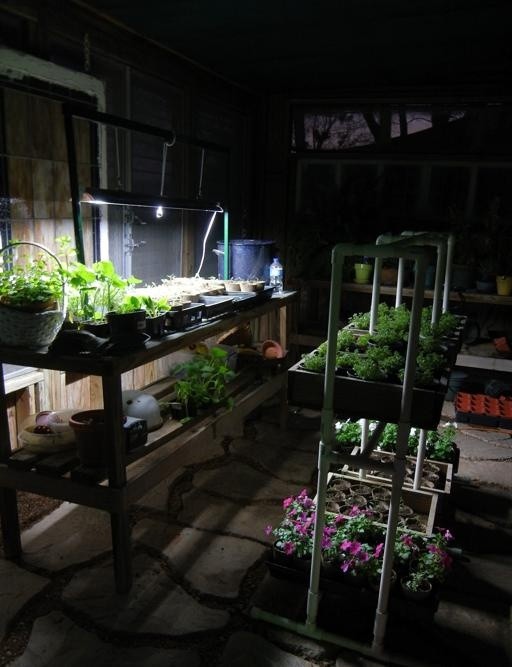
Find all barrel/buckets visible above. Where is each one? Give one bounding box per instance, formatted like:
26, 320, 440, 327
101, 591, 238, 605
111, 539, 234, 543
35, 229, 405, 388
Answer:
212, 238, 273, 285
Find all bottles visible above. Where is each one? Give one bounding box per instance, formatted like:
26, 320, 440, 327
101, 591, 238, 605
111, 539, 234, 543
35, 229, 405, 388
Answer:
269, 258, 283, 294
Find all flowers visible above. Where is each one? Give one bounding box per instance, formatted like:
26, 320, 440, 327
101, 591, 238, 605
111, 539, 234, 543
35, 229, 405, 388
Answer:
265, 490, 453, 586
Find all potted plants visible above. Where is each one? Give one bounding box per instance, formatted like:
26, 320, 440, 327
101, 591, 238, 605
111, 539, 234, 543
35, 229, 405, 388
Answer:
68, 250, 142, 339
142, 295, 170, 338
129, 296, 145, 334
287, 331, 457, 431
107, 303, 148, 350
342, 302, 467, 341
171, 346, 235, 423
335, 419, 458, 474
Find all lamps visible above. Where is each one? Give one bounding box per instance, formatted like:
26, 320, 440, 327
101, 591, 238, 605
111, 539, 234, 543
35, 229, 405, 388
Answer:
156, 137, 174, 220
80, 190, 229, 212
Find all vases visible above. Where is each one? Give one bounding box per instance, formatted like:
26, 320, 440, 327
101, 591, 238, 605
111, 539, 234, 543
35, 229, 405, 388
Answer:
353, 263, 372, 283
382, 264, 398, 286
497, 276, 512, 296
413, 266, 434, 289
140, 281, 267, 310
69, 409, 126, 462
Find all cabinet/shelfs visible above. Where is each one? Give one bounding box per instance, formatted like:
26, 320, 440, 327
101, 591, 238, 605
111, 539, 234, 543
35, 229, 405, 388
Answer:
315, 277, 511, 304
0, 294, 296, 588
456, 353, 509, 372
